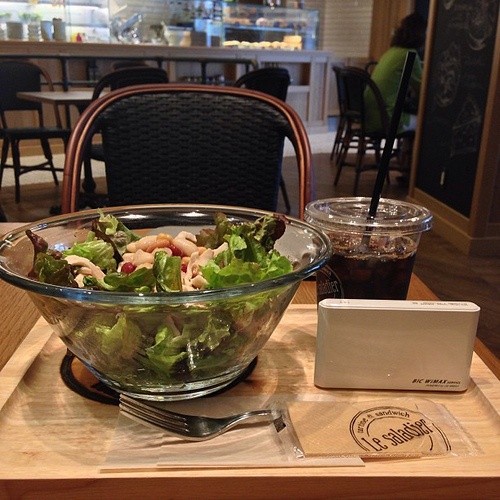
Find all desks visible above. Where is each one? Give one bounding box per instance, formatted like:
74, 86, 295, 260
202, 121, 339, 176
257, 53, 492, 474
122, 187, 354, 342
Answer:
16, 89, 92, 129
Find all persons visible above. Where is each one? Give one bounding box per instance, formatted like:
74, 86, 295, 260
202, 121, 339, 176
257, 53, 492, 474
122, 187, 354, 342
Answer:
365, 13, 426, 192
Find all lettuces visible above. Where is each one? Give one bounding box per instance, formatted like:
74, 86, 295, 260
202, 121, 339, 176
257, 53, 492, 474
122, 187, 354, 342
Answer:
26, 209, 293, 384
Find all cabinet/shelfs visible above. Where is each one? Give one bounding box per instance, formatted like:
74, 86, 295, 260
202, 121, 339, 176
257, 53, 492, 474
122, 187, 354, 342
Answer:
0, 40, 333, 157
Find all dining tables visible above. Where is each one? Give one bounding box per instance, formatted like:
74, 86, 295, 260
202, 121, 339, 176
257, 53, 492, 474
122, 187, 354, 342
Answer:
0, 224, 500, 500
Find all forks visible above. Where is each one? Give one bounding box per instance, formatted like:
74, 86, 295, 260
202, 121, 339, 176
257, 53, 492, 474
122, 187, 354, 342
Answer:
119, 394, 287, 441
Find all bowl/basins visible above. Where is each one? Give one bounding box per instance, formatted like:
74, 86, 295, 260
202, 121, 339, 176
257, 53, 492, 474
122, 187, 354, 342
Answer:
0, 204, 333, 401
164, 27, 184, 47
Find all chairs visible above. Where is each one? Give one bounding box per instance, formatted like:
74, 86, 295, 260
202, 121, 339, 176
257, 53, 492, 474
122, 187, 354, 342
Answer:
0, 58, 417, 221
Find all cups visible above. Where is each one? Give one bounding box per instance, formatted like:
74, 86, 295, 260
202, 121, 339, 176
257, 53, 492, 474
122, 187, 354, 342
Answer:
6, 22, 23, 40
40, 20, 52, 40
305, 197, 436, 314
284, 36, 301, 50
54, 22, 65, 40
195, 19, 222, 48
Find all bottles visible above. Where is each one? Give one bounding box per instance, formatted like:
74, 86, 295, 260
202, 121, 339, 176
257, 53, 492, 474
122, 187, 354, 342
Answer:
71, 33, 86, 42
